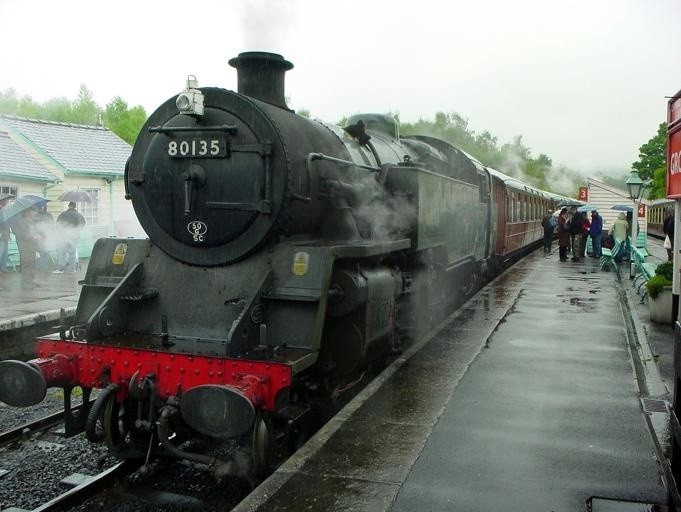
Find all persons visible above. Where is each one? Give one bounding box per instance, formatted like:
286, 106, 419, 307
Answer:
0, 187, 93, 284
541, 198, 635, 262
663, 208, 675, 262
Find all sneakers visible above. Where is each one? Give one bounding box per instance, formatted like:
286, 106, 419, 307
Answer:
587, 252, 601, 258
560, 249, 579, 262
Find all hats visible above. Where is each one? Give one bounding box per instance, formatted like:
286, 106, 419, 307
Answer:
591, 208, 596, 212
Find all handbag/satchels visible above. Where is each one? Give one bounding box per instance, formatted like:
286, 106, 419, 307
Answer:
664, 235, 671, 249
603, 235, 613, 248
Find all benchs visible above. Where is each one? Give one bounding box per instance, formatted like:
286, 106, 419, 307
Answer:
586, 231, 663, 304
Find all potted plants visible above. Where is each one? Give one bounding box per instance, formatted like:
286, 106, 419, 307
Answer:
646, 259, 674, 324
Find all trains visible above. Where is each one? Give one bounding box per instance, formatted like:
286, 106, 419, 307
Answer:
1, 46, 587, 485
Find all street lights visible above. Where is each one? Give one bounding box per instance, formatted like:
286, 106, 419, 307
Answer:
624, 168, 643, 279
